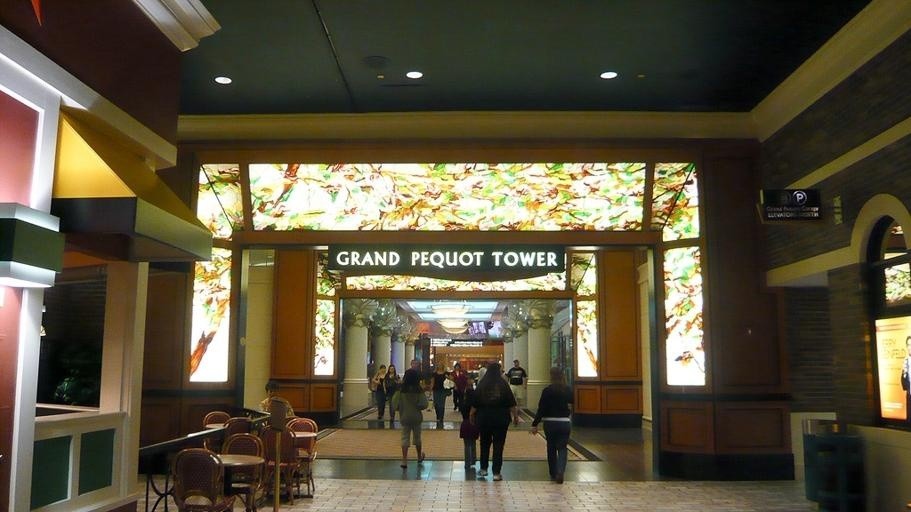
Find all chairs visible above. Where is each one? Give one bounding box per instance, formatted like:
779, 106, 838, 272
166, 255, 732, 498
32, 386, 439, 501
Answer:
166, 410, 319, 512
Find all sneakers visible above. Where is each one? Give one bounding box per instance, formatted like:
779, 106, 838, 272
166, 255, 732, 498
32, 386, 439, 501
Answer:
465, 457, 475, 468
378, 413, 394, 422
454, 403, 458, 410
437, 416, 443, 420
478, 469, 488, 476
493, 474, 502, 480
552, 474, 563, 483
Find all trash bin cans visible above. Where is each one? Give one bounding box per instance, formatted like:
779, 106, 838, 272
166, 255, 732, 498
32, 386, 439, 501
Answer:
801, 419, 866, 512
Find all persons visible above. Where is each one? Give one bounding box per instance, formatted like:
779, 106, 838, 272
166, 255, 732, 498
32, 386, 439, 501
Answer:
410, 359, 528, 432
372, 365, 387, 423
257, 382, 304, 479
392, 369, 428, 468
530, 366, 574, 484
901, 337, 911, 427
459, 391, 479, 468
382, 365, 401, 424
469, 363, 520, 481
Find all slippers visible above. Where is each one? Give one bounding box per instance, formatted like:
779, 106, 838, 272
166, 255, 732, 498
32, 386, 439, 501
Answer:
400, 465, 407, 468
418, 452, 425, 462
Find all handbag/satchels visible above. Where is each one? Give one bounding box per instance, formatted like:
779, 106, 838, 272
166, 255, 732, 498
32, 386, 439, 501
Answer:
443, 379, 455, 389
